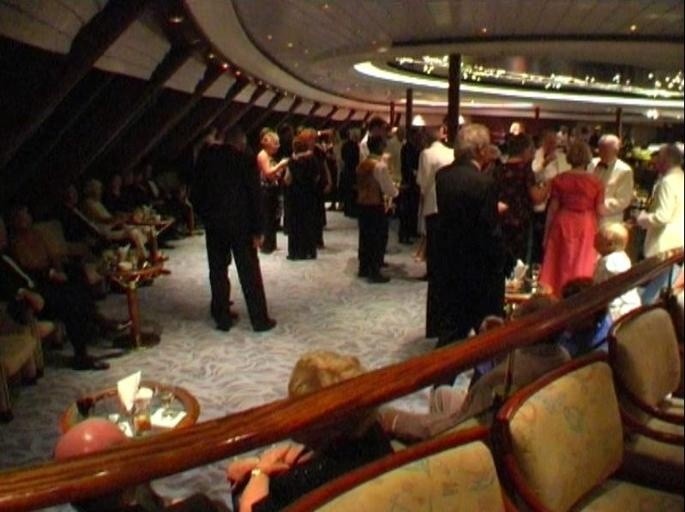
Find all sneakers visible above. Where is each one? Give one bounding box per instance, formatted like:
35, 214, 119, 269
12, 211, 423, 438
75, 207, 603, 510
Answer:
215, 312, 278, 334
356, 261, 391, 285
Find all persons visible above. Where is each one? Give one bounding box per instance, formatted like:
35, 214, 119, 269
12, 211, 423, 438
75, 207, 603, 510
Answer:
55, 293, 572, 511
193, 113, 684, 391
0, 162, 194, 371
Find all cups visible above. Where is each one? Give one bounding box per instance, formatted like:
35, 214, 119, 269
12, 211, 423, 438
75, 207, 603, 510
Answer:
158, 383, 175, 415
131, 399, 154, 438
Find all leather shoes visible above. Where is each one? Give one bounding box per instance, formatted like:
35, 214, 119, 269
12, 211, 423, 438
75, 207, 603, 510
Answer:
96, 317, 133, 338
70, 353, 111, 372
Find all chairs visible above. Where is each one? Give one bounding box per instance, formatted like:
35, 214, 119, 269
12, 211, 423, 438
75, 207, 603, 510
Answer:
37, 219, 67, 263
0, 302, 46, 421
